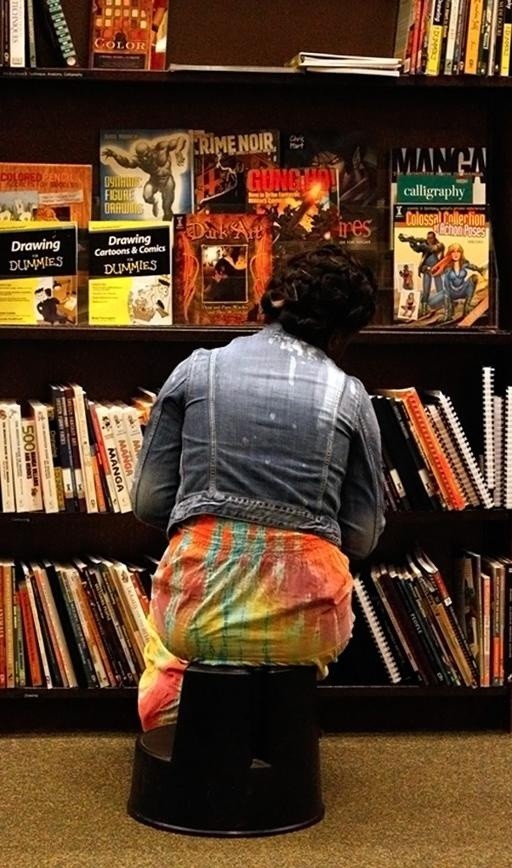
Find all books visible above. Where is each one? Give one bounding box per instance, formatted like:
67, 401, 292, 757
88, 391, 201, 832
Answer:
87, 125, 494, 331
0, 162, 93, 325
354, 551, 512, 691
0, 383, 160, 513
369, 367, 512, 510
1, 0, 169, 71
392, 0, 511, 77
170, 52, 401, 78
0, 554, 159, 688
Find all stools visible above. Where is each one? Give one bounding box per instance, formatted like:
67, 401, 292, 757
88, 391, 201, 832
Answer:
126, 661, 324, 838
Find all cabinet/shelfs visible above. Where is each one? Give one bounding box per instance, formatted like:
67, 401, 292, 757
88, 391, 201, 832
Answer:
1, 67, 512, 736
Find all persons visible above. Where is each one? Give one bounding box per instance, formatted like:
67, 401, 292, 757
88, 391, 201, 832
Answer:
133, 246, 386, 730
